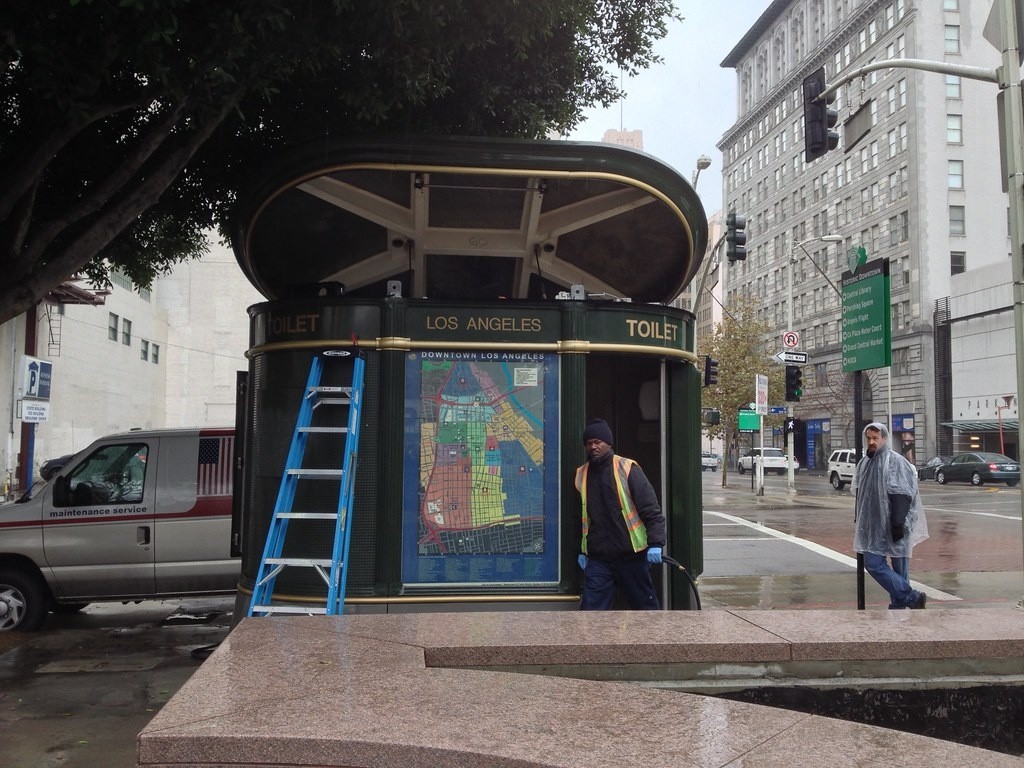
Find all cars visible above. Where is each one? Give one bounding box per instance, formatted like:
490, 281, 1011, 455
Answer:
918, 452, 1021, 487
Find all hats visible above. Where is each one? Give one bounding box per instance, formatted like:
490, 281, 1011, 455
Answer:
581, 416, 614, 449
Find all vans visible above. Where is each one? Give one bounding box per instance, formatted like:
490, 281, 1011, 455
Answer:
0, 427, 243, 631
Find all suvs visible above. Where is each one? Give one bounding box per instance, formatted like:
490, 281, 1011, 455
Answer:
737, 448, 800, 476
826, 448, 866, 490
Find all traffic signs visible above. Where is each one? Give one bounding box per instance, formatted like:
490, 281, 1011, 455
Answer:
770, 351, 808, 366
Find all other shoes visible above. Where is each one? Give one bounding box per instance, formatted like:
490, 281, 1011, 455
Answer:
914, 592, 926, 609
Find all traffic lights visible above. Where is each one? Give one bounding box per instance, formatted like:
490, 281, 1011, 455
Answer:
704, 358, 718, 386
785, 365, 802, 402
803, 66, 839, 163
706, 411, 720, 425
786, 418, 796, 433
726, 207, 747, 267
784, 234, 843, 495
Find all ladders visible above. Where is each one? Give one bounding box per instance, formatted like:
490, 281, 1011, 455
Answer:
246, 349, 367, 615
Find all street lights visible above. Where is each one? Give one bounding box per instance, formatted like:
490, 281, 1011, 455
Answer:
692, 157, 712, 193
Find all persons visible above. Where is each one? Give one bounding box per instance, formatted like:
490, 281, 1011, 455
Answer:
567, 417, 665, 610
856, 423, 929, 610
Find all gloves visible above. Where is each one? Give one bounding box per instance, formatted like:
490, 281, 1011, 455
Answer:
646, 547, 663, 564
578, 552, 588, 571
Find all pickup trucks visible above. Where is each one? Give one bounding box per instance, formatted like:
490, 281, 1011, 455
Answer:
702, 454, 717, 472
